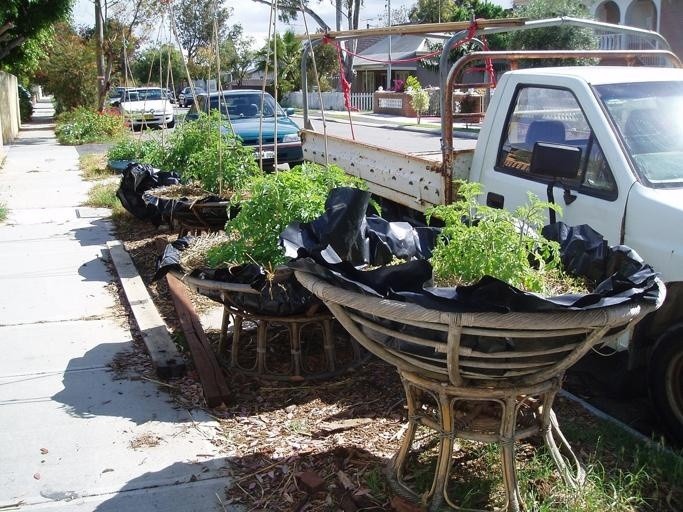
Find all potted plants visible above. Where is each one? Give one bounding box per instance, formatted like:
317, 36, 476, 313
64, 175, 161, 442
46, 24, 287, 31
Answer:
105, 105, 666, 512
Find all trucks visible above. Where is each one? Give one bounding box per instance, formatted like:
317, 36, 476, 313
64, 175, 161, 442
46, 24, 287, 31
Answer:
301, 49, 682, 434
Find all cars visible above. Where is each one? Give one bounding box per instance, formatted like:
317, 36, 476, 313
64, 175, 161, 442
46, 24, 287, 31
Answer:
104, 87, 175, 131
182, 89, 304, 173
178, 87, 206, 107
